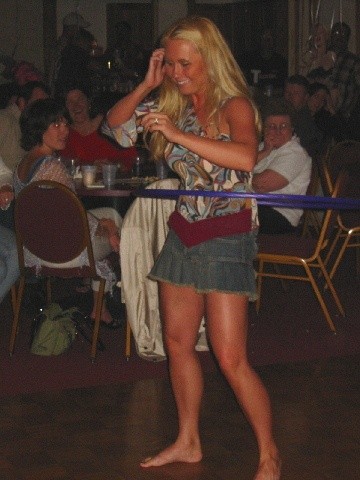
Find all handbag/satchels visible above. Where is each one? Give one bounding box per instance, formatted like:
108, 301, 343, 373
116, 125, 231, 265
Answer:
28, 300, 106, 358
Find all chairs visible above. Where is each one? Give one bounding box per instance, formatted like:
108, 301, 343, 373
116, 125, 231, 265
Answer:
119, 178, 210, 362
7, 180, 133, 362
254, 139, 360, 332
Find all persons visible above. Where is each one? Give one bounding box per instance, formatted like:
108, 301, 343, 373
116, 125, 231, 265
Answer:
12, 99, 124, 330
103, 17, 283, 480
55, 83, 138, 176
0, 159, 26, 304
0, 81, 49, 172
37, 12, 148, 122
251, 110, 313, 236
238, 21, 360, 159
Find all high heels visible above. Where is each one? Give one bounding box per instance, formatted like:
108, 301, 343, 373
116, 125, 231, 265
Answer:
90, 317, 123, 330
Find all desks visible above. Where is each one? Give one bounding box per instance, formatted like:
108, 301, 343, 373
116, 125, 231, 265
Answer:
75, 188, 134, 198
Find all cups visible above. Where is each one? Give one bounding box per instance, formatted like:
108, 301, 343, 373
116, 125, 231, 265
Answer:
53, 151, 64, 163
67, 159, 81, 177
81, 165, 97, 187
101, 164, 118, 186
129, 152, 144, 177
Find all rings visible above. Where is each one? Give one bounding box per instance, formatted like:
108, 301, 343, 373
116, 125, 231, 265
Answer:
154, 117, 158, 124
5, 199, 9, 201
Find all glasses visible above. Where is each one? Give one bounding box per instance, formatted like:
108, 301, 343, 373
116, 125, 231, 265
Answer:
48, 122, 70, 128
332, 30, 350, 37
265, 122, 290, 129
